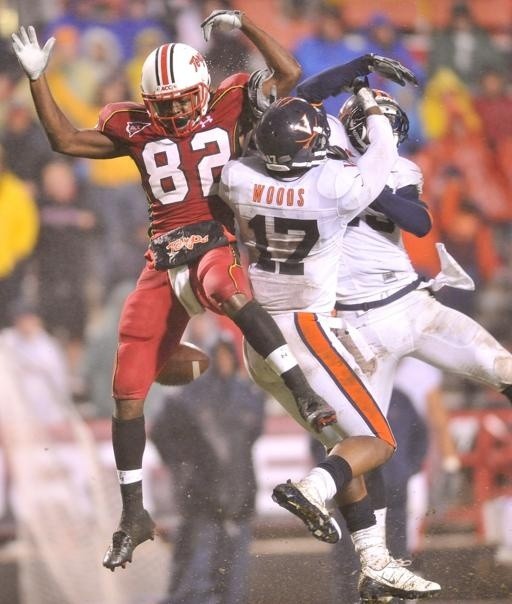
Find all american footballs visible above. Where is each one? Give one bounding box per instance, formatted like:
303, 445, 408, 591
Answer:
155, 341, 211, 385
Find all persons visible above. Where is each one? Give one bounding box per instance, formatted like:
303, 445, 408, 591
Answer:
0, 0, 512, 604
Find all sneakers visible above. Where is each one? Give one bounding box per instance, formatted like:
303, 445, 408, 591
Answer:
359, 556, 441, 604
272, 479, 342, 544
300, 395, 337, 430
103, 510, 156, 571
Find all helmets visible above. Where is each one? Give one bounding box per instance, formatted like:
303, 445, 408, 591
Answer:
338, 89, 409, 154
256, 96, 325, 172
141, 43, 211, 136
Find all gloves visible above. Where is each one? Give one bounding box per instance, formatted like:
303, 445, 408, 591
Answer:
201, 10, 245, 41
371, 53, 418, 86
247, 69, 277, 119
12, 25, 55, 80
